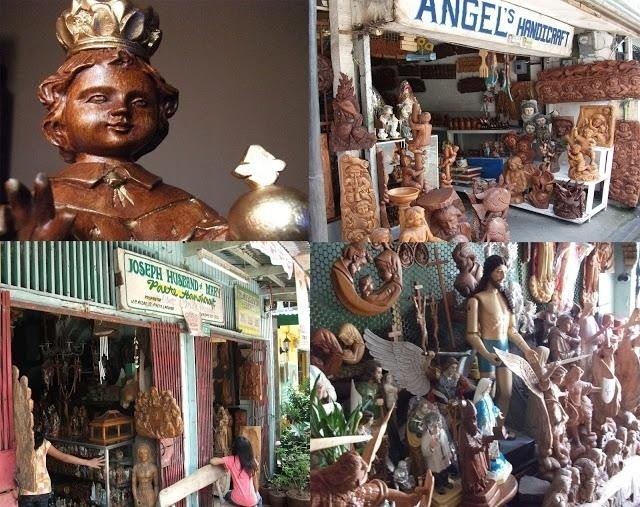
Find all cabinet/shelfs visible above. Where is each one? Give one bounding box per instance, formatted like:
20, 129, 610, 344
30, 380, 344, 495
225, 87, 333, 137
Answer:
43, 433, 133, 507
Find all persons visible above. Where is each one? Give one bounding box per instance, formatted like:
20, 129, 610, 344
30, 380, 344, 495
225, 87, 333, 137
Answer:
0, 0, 228, 238
312, 55, 640, 507
210, 436, 264, 507
20, 428, 108, 505
129, 442, 159, 506
